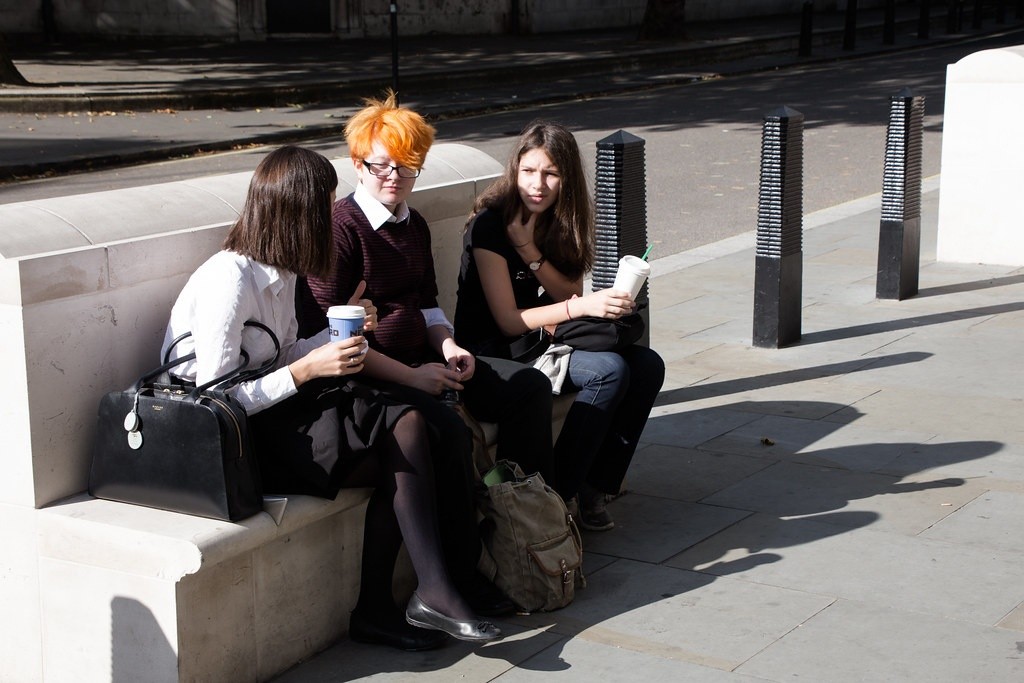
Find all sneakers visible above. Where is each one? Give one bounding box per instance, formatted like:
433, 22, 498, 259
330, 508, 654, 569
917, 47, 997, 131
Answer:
577, 488, 614, 530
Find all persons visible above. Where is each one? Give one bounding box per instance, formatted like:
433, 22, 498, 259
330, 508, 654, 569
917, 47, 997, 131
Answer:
160, 146, 503, 649
453, 124, 668, 530
293, 97, 553, 616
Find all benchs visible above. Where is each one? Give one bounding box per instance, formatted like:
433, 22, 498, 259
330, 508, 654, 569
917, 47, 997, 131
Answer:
1, 142, 632, 683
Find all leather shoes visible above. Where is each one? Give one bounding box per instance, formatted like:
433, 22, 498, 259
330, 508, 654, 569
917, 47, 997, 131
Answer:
405, 594, 503, 641
349, 613, 446, 651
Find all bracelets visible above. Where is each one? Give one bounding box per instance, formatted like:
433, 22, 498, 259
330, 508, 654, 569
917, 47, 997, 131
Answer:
565, 299, 571, 320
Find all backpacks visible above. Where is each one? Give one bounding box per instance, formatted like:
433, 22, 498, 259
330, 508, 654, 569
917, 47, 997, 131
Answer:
478, 460, 586, 612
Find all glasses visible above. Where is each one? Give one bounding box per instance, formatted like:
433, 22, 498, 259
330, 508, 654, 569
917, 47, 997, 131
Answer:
362, 161, 420, 178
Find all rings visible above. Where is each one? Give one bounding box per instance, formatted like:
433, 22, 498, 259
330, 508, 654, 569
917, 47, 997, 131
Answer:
351, 358, 355, 366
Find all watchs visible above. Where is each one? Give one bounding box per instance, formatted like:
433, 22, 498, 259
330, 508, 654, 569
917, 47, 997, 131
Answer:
528, 255, 546, 271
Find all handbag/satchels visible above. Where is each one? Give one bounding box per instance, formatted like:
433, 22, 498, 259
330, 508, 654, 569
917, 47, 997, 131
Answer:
89, 381, 264, 522
552, 314, 645, 352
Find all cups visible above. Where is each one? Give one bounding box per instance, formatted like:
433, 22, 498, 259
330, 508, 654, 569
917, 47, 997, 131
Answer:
614, 255, 652, 308
327, 304, 367, 342
